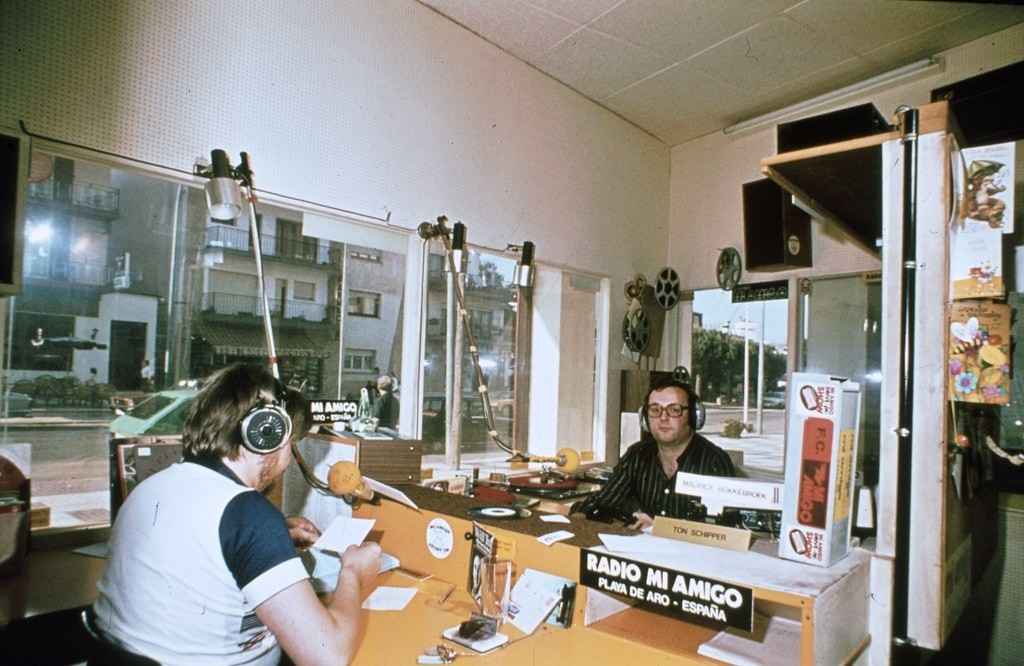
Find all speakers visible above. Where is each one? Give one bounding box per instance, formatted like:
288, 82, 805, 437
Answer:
743, 177, 812, 273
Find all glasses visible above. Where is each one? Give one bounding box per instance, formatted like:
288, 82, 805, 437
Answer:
646, 400, 693, 426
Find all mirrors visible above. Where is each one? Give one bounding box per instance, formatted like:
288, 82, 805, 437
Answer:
0, 117, 426, 540
451, 240, 529, 470
675, 274, 797, 411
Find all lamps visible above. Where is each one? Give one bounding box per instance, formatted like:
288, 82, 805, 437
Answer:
196, 149, 243, 222
722, 55, 946, 139
418, 214, 466, 272
506, 240, 536, 287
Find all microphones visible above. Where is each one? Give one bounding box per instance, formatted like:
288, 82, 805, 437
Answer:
523, 448, 580, 472
328, 461, 382, 507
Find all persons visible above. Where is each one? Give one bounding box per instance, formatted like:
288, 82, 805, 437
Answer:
141, 360, 154, 394
369, 368, 398, 431
96, 361, 383, 665
569, 381, 740, 545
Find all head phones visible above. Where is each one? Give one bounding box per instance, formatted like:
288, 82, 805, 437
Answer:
239, 383, 293, 455
639, 401, 705, 432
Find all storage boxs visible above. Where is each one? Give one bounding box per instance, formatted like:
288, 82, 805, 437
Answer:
778, 370, 861, 567
